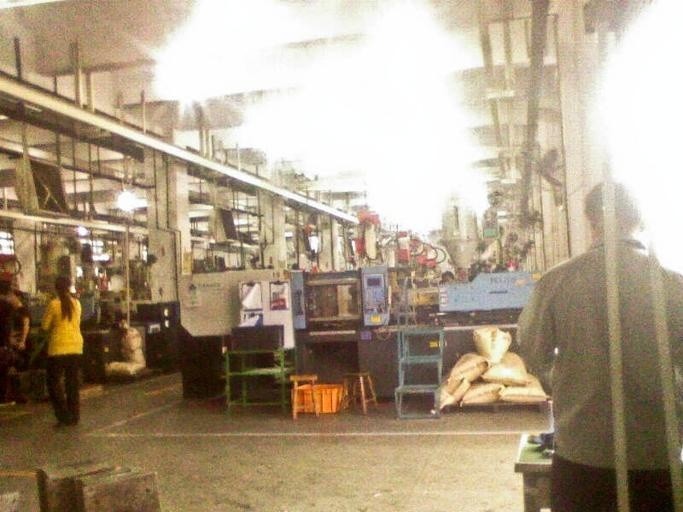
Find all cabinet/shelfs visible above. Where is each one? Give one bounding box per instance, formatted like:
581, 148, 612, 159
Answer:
224, 345, 293, 413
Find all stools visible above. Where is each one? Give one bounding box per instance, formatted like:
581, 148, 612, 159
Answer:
290, 374, 321, 420
343, 371, 379, 414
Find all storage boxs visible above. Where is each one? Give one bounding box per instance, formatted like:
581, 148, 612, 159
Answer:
35, 462, 117, 511
74, 467, 162, 511
291, 384, 342, 413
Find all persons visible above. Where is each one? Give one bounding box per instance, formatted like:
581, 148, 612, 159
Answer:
5, 289, 35, 374
515, 176, 682, 512
40, 277, 83, 427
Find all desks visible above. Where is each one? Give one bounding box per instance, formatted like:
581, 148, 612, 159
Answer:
513, 433, 557, 512
